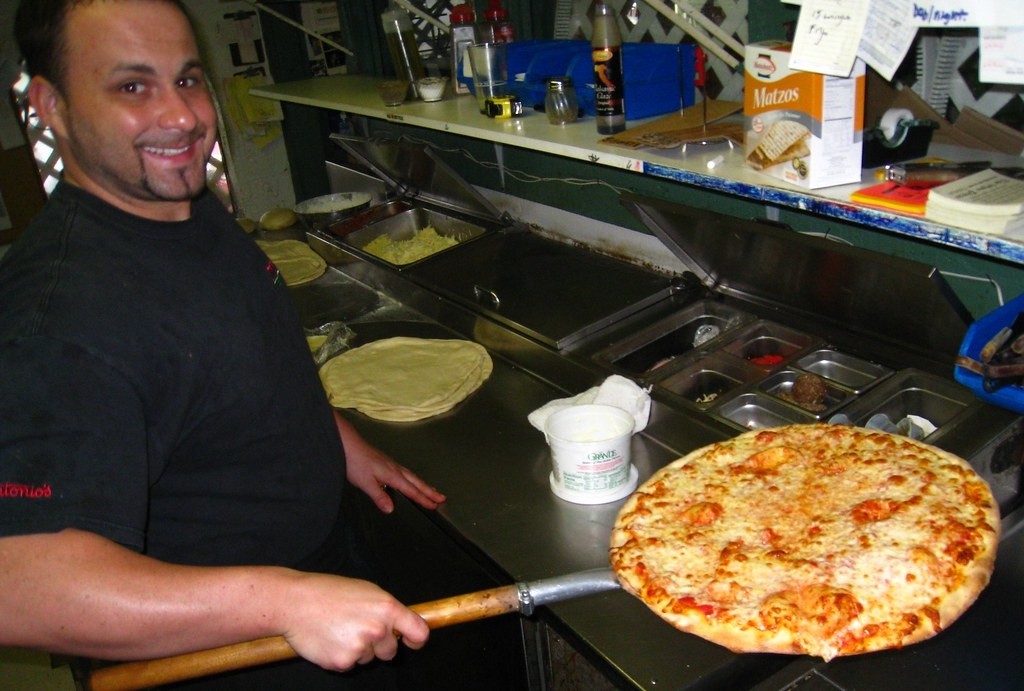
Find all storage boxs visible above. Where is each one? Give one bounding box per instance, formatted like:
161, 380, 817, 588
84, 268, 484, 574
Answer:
955, 106, 1024, 155
745, 41, 865, 189
457, 38, 694, 123
891, 86, 999, 152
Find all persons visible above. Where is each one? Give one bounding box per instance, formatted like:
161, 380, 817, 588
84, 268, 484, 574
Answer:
0, 0, 445, 691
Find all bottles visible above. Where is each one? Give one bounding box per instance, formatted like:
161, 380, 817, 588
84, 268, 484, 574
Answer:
449, 4, 479, 97
546, 78, 578, 124
482, 9, 515, 44
592, 4, 626, 135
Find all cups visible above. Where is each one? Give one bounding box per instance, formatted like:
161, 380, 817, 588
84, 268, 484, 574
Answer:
467, 44, 508, 113
380, 9, 429, 98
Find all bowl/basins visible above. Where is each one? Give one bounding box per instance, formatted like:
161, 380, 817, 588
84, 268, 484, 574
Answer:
415, 76, 449, 102
293, 191, 371, 228
543, 404, 635, 498
377, 81, 410, 105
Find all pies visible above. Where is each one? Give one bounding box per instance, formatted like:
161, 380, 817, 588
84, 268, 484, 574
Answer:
319, 336, 493, 423
256, 240, 325, 285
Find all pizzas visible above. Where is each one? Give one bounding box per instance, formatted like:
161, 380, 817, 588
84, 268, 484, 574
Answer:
609, 427, 1000, 659
